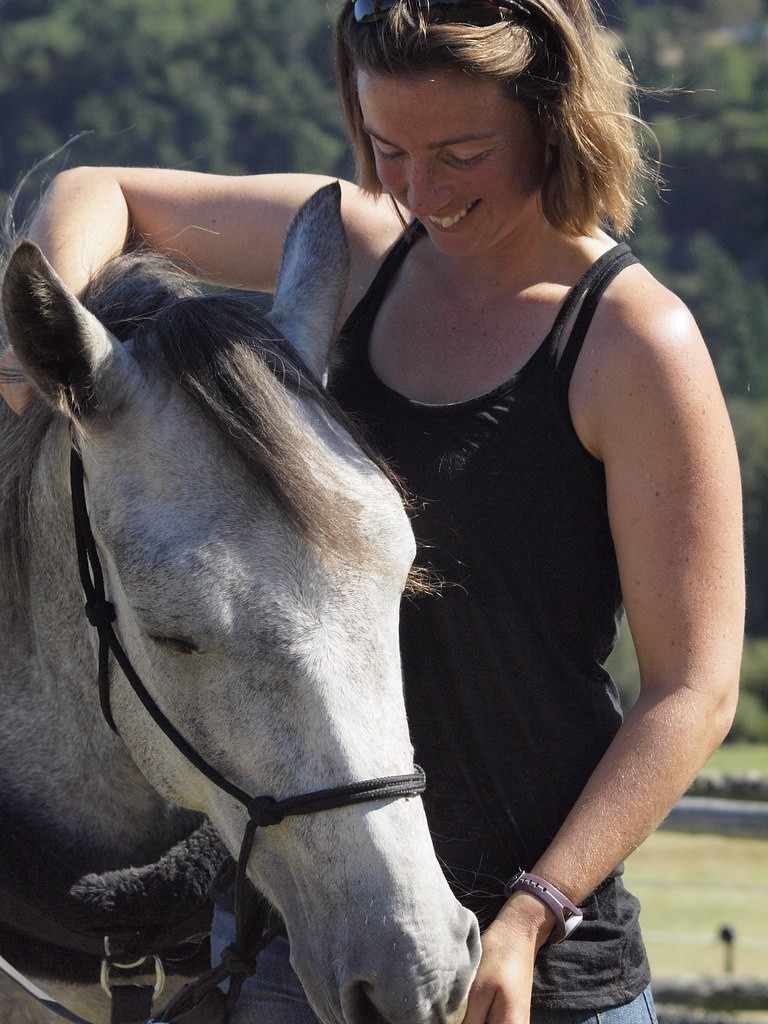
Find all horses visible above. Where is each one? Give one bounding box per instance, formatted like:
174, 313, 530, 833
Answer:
0, 239, 483, 1023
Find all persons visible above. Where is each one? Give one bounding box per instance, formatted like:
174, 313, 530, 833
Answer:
1, 0, 747, 1024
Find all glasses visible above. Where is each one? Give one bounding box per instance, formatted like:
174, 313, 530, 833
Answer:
352, 0, 538, 27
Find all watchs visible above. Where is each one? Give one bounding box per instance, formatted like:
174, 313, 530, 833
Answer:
499, 862, 582, 949
